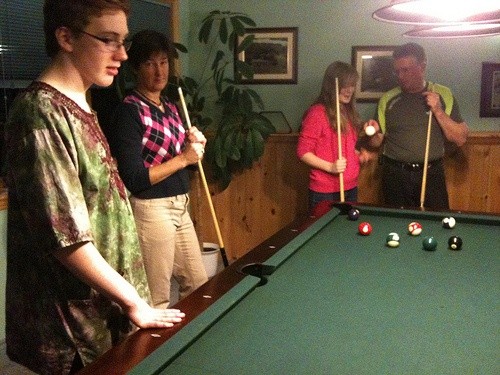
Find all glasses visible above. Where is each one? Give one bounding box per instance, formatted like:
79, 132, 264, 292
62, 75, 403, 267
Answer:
57, 24, 133, 52
391, 64, 419, 76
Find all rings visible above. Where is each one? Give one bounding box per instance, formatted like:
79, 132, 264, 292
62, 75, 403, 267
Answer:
201, 151, 203, 153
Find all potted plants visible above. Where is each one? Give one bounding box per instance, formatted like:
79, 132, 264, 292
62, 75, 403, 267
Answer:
161, 9, 276, 280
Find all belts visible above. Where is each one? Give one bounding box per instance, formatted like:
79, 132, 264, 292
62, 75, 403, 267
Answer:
383, 155, 443, 169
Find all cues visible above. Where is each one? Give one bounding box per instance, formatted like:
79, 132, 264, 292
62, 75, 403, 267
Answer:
177, 86, 229, 268
419, 81, 436, 211
336, 76, 345, 203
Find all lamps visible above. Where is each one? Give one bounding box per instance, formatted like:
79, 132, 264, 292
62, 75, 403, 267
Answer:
372, 0, 500, 39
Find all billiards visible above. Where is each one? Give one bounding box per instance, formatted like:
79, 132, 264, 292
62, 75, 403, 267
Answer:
365, 125, 377, 137
448, 235, 463, 250
422, 234, 438, 251
347, 207, 360, 221
442, 215, 456, 229
408, 221, 423, 236
385, 231, 400, 248
358, 221, 373, 236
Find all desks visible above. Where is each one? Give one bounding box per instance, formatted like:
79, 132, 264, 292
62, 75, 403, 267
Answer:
74, 201, 500, 375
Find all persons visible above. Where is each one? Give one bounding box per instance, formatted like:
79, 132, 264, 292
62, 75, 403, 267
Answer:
364, 43, 466, 208
107, 30, 207, 309
5, 0, 185, 375
297, 61, 367, 209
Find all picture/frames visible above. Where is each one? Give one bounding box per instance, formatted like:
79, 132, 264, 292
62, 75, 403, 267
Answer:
234, 27, 298, 84
479, 62, 500, 118
351, 45, 399, 103
